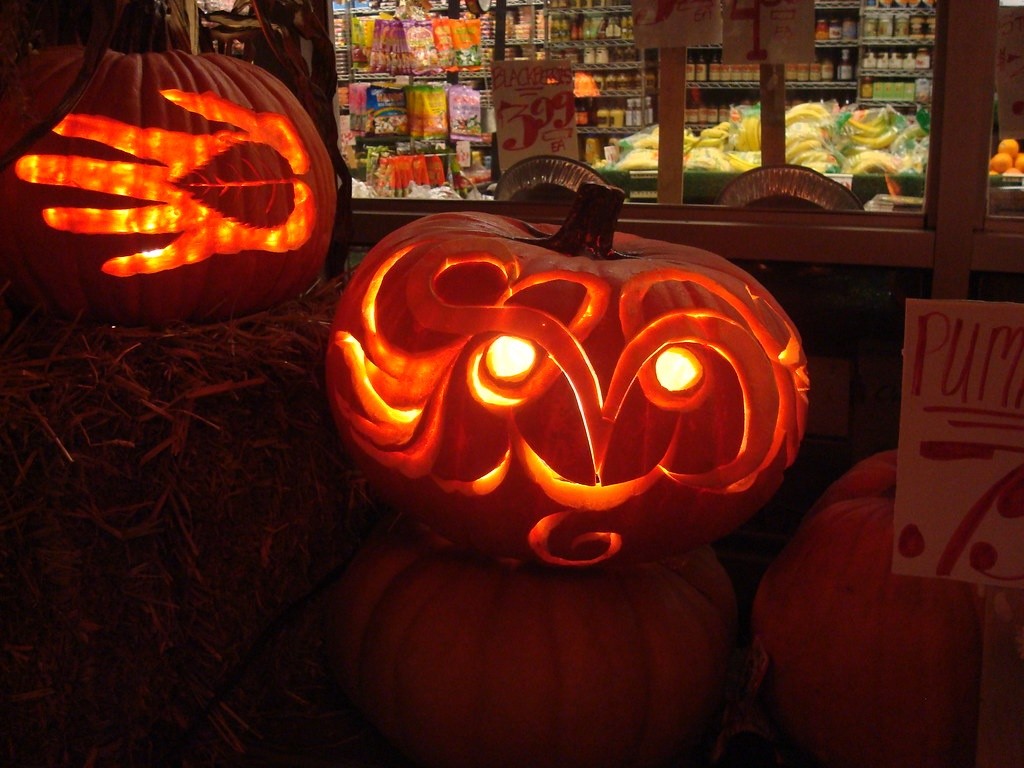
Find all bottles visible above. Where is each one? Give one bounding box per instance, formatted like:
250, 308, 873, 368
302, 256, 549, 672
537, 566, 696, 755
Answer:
627, 98, 654, 127
683, 49, 850, 125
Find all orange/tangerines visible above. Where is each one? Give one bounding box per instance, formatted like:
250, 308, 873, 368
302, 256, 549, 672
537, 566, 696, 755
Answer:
988, 139, 1024, 177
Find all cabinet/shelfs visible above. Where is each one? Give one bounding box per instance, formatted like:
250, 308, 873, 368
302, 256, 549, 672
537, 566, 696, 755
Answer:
683, 0, 935, 131
331, 0, 659, 201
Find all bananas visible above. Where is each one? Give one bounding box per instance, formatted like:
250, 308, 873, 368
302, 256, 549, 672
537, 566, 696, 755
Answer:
632, 102, 898, 174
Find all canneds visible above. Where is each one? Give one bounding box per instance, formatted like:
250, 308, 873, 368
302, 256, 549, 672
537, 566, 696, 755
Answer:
814, 15, 857, 42
861, 13, 936, 70
860, 76, 873, 98
584, 46, 609, 64
